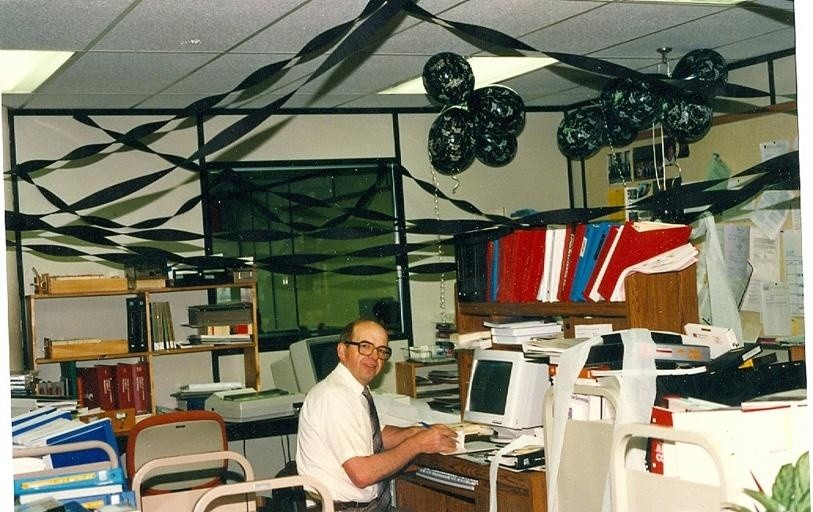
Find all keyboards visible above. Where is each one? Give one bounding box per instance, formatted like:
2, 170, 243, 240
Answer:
416, 467, 479, 492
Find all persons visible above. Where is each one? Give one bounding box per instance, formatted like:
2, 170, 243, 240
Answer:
295, 319, 458, 512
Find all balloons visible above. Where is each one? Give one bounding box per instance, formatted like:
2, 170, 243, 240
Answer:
558, 49, 728, 161
423, 51, 525, 173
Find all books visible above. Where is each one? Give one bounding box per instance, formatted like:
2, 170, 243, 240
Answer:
126, 297, 177, 355
10, 371, 68, 399
181, 383, 242, 391
450, 316, 563, 352
177, 303, 253, 349
10, 399, 136, 511
59, 361, 151, 414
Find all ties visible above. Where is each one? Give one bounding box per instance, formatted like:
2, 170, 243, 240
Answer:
363, 386, 391, 511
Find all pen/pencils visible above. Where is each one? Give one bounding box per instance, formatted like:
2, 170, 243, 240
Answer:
421, 422, 460, 443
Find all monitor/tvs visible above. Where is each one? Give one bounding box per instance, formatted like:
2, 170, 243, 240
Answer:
462, 349, 550, 443
270, 334, 341, 396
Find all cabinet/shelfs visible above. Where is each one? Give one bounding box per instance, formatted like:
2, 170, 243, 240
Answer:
396, 361, 460, 415
454, 256, 698, 422
25, 282, 300, 456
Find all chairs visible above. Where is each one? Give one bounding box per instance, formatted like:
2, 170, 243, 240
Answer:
126, 410, 229, 496
286, 461, 340, 512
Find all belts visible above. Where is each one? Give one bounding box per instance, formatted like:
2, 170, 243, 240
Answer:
334, 500, 368, 511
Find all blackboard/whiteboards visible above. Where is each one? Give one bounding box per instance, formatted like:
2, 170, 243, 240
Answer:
581, 100, 805, 349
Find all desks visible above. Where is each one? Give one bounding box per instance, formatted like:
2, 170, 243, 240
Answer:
395, 449, 548, 512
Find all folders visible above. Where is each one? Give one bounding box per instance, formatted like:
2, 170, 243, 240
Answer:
484, 447, 546, 471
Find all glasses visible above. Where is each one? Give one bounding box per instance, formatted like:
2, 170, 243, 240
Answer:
344, 340, 392, 360
